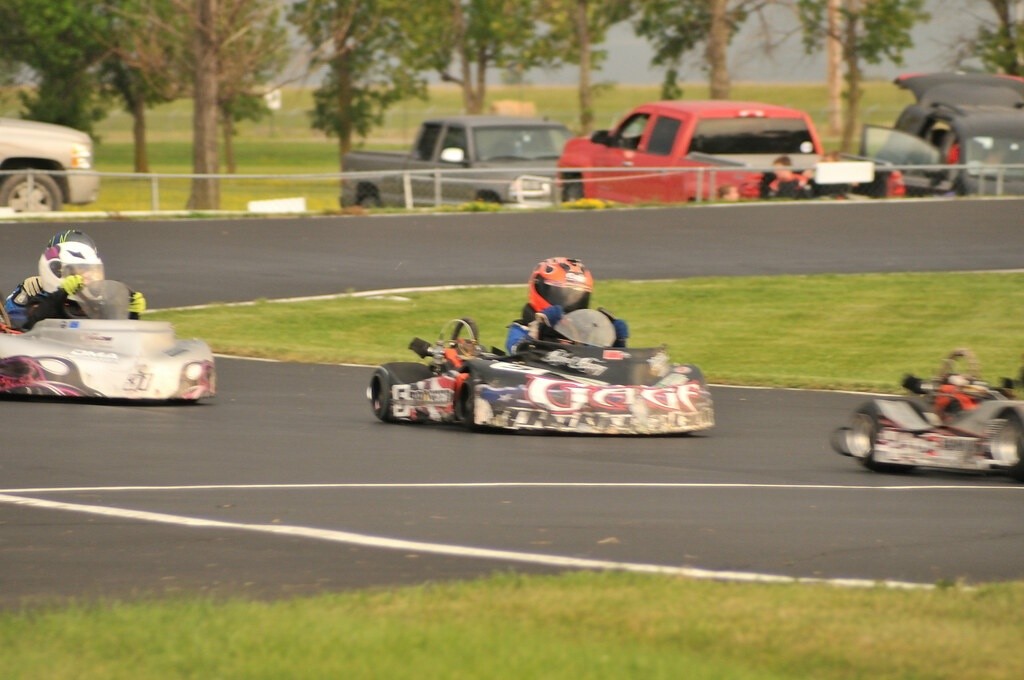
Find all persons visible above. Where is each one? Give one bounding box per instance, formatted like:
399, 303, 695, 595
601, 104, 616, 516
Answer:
767, 156, 865, 201
718, 184, 740, 201
506, 257, 629, 360
4, 230, 146, 333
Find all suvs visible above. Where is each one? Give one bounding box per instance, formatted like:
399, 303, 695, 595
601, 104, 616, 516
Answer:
860, 71, 1024, 196
0, 116, 98, 210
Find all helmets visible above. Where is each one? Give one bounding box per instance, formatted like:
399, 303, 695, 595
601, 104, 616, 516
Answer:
38, 242, 104, 304
528, 257, 592, 319
48, 229, 98, 256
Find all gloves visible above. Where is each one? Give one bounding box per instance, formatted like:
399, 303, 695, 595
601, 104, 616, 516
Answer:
12, 277, 45, 306
126, 292, 146, 313
535, 305, 561, 328
614, 319, 628, 341
57, 275, 84, 296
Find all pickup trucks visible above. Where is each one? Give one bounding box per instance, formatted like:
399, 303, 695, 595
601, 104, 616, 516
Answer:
338, 114, 576, 207
557, 100, 905, 204
